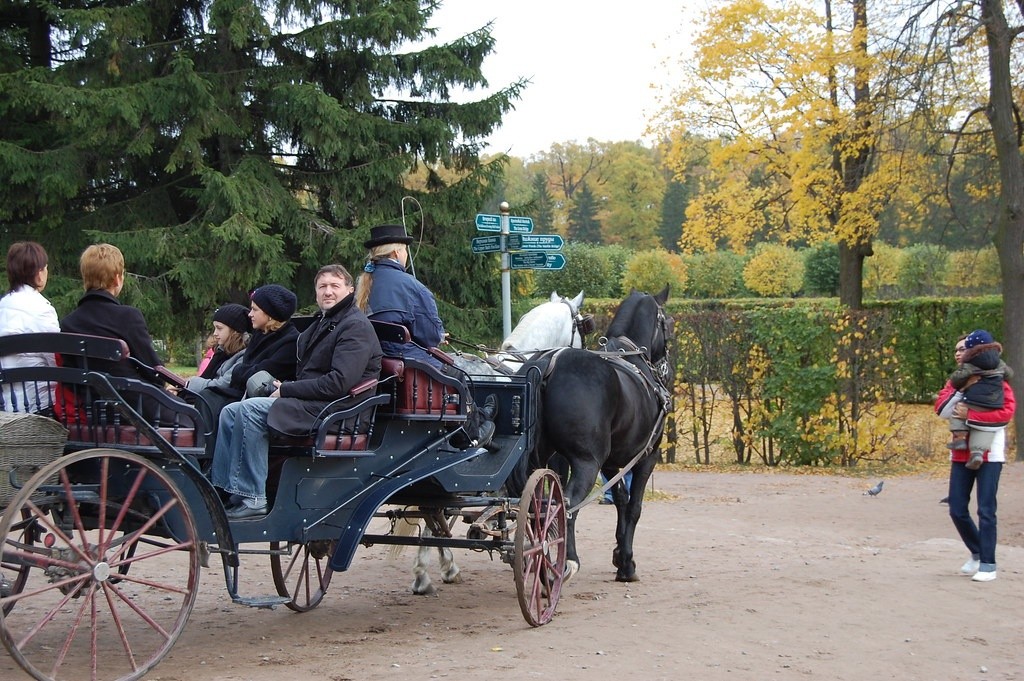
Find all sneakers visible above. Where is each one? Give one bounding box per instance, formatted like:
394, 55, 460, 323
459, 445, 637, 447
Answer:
962, 557, 980, 572
946, 436, 967, 449
965, 451, 983, 470
972, 571, 997, 581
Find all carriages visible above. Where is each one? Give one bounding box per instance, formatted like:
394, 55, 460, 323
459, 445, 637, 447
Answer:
1, 273, 675, 681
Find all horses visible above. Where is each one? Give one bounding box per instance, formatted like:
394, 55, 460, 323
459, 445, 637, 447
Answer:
387, 281, 674, 596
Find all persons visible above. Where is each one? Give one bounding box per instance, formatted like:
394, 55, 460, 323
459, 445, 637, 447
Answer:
0, 240, 58, 417
54, 243, 194, 428
933, 333, 1017, 582
354, 225, 499, 449
951, 328, 1015, 470
169, 283, 302, 480
212, 266, 382, 520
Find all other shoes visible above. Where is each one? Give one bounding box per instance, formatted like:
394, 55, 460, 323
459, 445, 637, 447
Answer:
599, 498, 613, 504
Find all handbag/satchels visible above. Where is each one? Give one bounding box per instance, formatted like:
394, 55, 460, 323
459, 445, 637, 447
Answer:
246, 371, 276, 397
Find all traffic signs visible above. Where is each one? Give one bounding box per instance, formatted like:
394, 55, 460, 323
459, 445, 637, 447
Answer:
471, 213, 566, 270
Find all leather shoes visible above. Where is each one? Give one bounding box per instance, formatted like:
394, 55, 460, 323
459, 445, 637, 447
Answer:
480, 394, 498, 421
225, 494, 268, 518
462, 421, 495, 462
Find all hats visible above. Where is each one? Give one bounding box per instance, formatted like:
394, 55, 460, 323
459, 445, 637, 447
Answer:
213, 303, 251, 333
250, 284, 297, 322
964, 329, 993, 349
363, 224, 413, 249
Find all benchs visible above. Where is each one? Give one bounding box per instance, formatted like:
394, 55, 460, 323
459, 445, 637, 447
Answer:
0, 316, 466, 458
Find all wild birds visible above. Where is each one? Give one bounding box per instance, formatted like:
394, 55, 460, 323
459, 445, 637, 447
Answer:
861, 480, 883, 498
